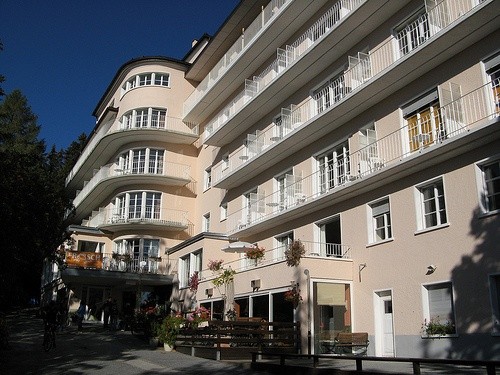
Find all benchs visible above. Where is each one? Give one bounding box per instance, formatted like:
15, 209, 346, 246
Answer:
321, 332, 369, 356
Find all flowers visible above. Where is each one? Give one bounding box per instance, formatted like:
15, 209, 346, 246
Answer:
206, 258, 224, 276
243, 244, 265, 265
285, 289, 302, 307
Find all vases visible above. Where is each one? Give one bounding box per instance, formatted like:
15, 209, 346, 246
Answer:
292, 301, 297, 304
250, 255, 257, 259
209, 265, 219, 270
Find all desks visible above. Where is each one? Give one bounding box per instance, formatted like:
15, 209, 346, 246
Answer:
369, 159, 384, 173
413, 134, 430, 150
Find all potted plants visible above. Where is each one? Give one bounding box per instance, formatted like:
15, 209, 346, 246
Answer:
420, 316, 456, 340
70, 314, 79, 330
143, 314, 183, 352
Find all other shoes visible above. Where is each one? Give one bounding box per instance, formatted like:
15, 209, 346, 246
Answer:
75, 330, 83, 334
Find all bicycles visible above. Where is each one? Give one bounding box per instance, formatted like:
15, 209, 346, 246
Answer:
42, 320, 56, 353
56, 316, 67, 336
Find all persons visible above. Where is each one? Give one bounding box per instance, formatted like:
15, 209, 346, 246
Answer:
57, 297, 69, 332
196, 304, 210, 319
74, 296, 160, 332
41, 300, 62, 349
